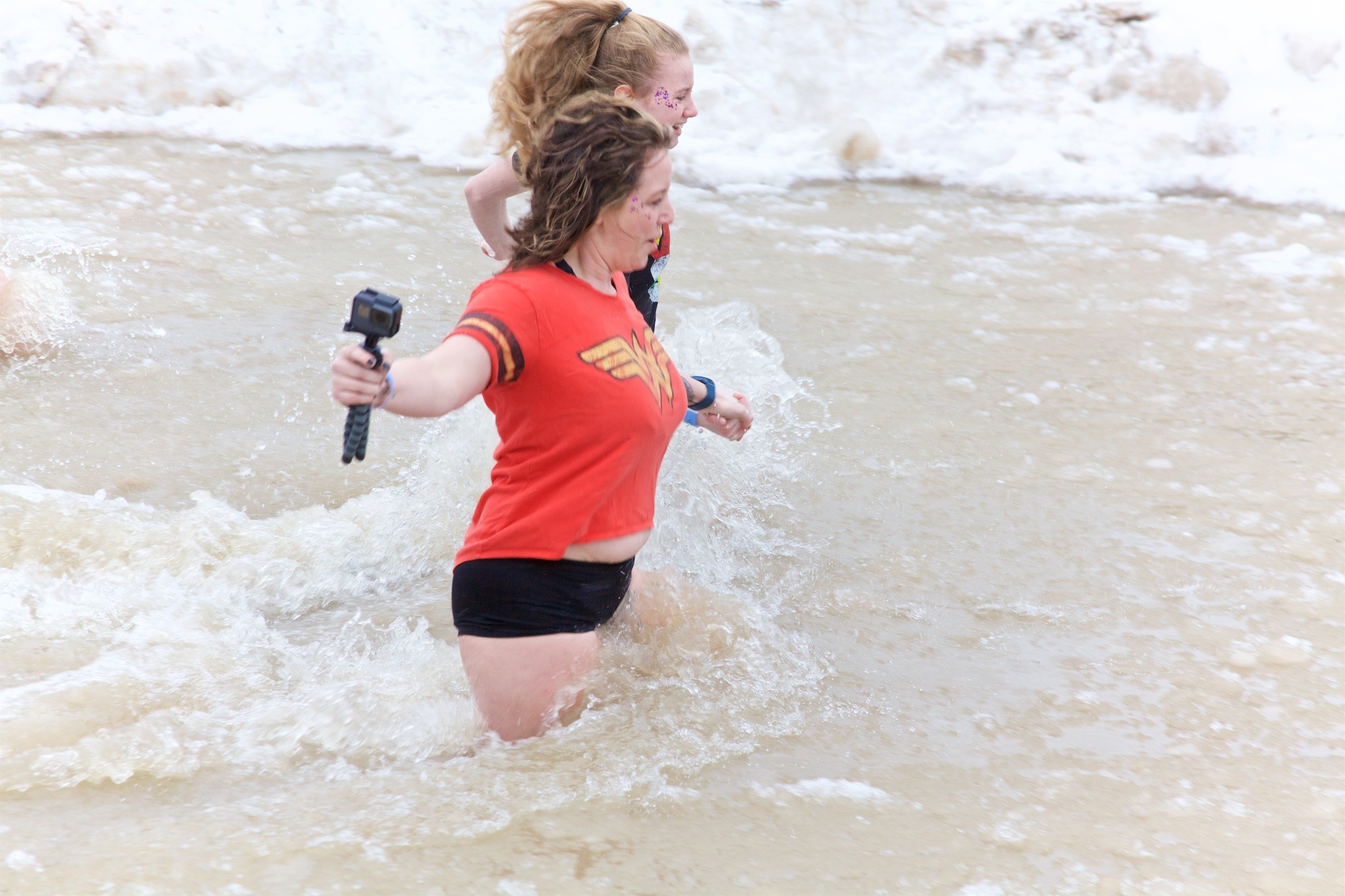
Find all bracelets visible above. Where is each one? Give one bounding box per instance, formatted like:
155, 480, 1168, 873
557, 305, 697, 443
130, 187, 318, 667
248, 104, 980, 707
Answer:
684, 409, 699, 427
375, 374, 394, 410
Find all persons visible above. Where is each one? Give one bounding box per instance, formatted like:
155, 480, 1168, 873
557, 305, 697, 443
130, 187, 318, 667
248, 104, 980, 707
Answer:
468, 0, 753, 443
332, 90, 754, 743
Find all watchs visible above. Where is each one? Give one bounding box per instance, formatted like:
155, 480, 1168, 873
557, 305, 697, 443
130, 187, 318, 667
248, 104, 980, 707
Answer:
689, 375, 716, 410
